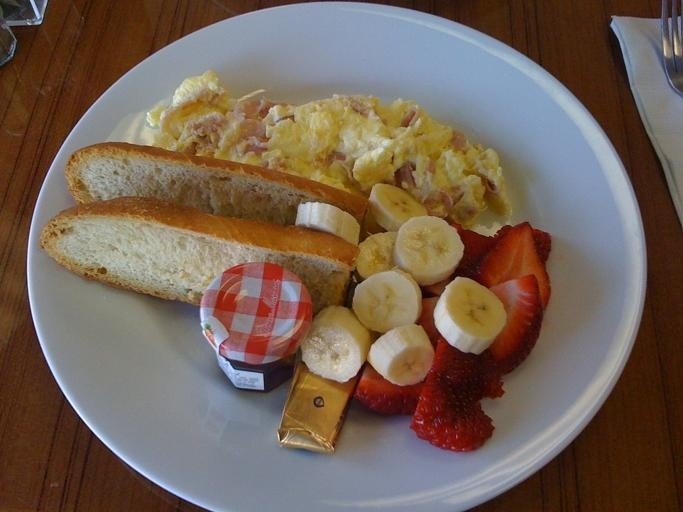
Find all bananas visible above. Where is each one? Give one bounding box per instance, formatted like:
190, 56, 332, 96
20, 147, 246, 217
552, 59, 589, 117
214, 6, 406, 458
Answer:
295, 182, 507, 386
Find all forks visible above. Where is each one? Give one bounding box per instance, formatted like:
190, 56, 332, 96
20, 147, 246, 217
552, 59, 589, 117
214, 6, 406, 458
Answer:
658, 2, 682, 99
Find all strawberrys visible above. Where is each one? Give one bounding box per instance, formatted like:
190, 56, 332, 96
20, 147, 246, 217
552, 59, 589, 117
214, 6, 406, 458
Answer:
354, 215, 553, 453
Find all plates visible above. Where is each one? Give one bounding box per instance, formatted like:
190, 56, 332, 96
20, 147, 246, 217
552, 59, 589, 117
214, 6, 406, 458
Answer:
26, 2, 650, 511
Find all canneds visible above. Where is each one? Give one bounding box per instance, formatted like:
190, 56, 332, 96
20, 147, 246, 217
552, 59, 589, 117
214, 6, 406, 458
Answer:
199, 260, 314, 394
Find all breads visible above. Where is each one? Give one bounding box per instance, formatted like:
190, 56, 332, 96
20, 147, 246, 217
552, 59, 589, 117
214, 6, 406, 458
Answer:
39, 140, 371, 316
